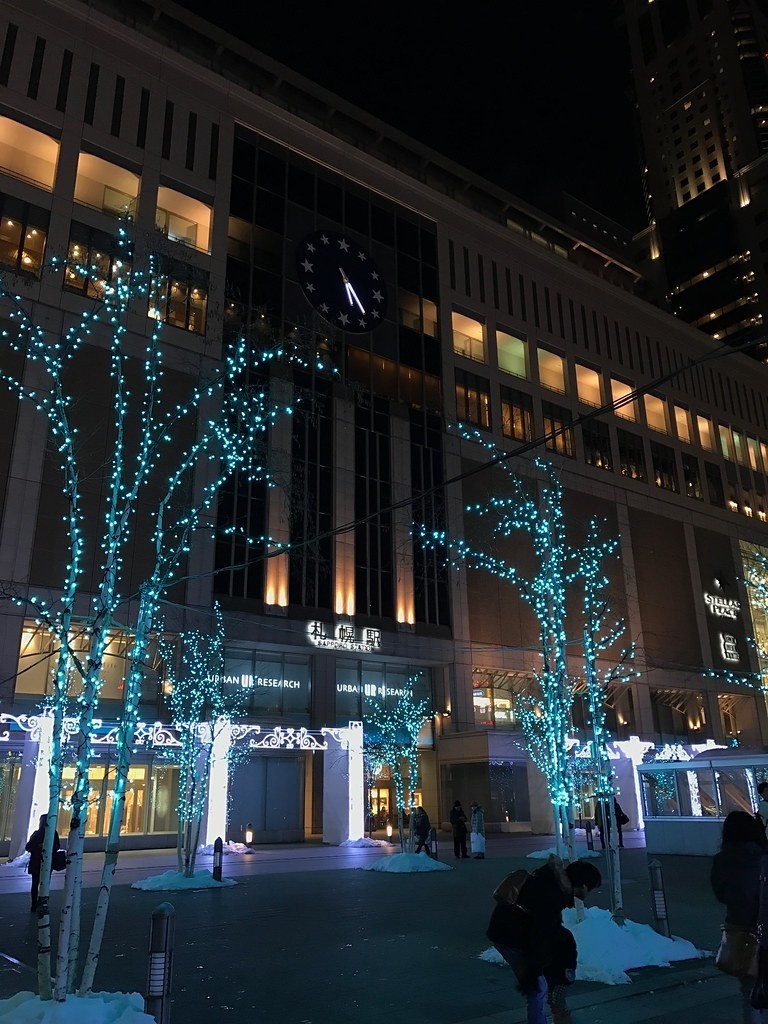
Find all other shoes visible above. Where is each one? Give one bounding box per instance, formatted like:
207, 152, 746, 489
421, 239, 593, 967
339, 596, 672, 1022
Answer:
462, 855, 470, 858
474, 856, 485, 859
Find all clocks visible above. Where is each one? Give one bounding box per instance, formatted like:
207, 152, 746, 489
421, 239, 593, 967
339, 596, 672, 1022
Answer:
298, 229, 387, 334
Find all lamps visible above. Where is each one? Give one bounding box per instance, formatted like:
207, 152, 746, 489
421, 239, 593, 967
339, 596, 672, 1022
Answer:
430, 840, 438, 852
587, 831, 593, 843
650, 889, 667, 919
213, 850, 223, 867
147, 951, 171, 996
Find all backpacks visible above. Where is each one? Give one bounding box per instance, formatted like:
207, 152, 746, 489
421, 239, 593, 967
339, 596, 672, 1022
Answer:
493, 868, 534, 912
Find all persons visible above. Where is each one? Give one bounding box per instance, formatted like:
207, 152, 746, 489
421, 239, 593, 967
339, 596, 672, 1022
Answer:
25, 814, 61, 913
710, 780, 768, 1024
470, 799, 486, 860
380, 807, 388, 822
450, 800, 470, 858
415, 807, 431, 856
486, 862, 601, 1024
594, 792, 630, 849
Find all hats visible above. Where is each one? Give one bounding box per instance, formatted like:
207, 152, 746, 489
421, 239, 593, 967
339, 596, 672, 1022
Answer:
469, 801, 478, 807
454, 800, 461, 807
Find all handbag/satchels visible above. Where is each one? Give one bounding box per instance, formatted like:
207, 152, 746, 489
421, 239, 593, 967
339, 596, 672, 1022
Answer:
618, 812, 629, 825
414, 834, 420, 842
51, 850, 67, 871
470, 832, 486, 853
713, 924, 765, 978
